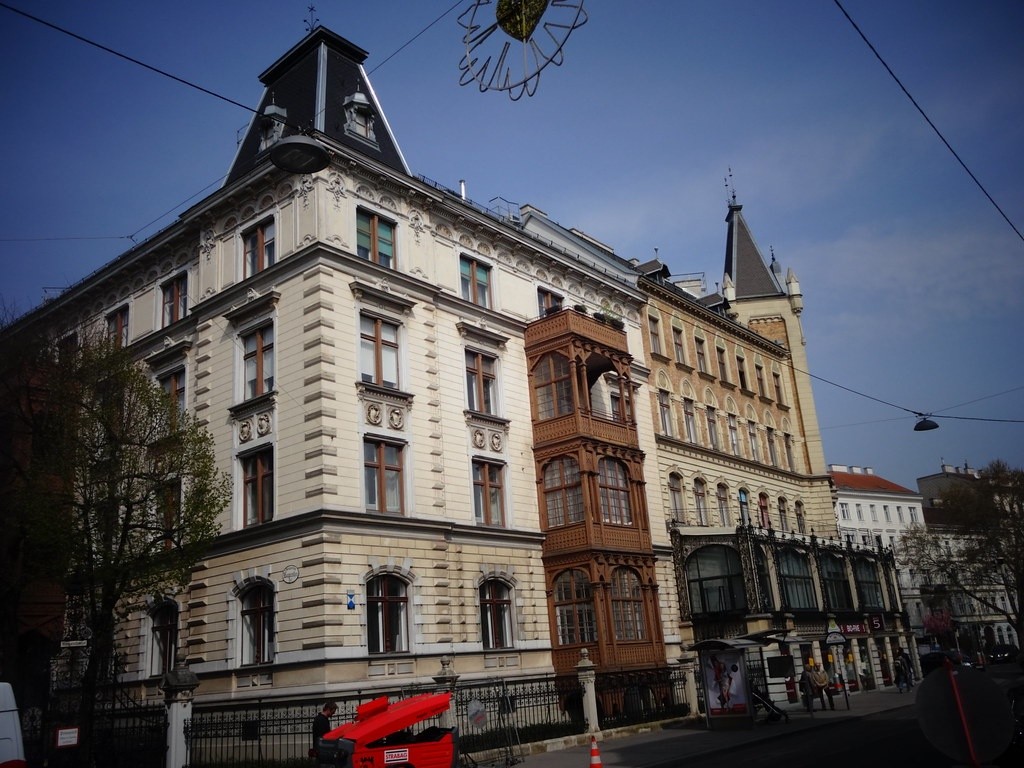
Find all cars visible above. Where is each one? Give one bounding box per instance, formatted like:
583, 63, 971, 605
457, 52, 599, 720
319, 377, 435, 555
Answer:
988, 644, 1019, 665
919, 653, 974, 680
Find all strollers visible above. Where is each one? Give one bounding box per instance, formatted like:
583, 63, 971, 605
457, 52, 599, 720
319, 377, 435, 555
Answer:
750, 682, 790, 726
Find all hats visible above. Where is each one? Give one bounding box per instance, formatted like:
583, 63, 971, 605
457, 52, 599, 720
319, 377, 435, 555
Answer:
816, 662, 821, 667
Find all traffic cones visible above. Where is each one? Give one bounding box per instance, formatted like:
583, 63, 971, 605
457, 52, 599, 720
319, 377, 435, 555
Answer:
589, 735, 604, 768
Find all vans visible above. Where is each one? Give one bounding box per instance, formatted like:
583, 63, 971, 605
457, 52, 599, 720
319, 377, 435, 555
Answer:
0, 679, 28, 768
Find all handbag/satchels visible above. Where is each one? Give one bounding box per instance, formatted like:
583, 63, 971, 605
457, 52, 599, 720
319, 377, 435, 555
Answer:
801, 690, 813, 707
798, 679, 807, 691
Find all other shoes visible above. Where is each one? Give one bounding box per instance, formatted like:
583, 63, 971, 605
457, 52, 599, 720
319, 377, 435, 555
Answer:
807, 708, 817, 712
822, 705, 826, 711
907, 690, 912, 692
831, 704, 835, 711
899, 688, 904, 694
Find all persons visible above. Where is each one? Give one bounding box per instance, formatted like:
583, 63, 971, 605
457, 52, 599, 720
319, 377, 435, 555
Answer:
709, 653, 735, 709
800, 663, 818, 711
892, 647, 919, 694
312, 700, 339, 768
810, 662, 837, 710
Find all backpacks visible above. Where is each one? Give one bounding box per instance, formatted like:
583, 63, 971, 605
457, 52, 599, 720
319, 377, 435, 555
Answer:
894, 658, 905, 675
